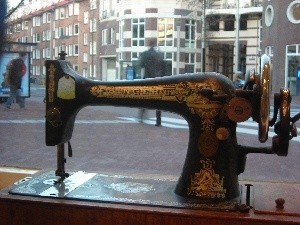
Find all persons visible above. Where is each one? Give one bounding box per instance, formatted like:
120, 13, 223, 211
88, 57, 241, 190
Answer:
231, 71, 246, 90
4, 51, 27, 111
132, 39, 165, 127
